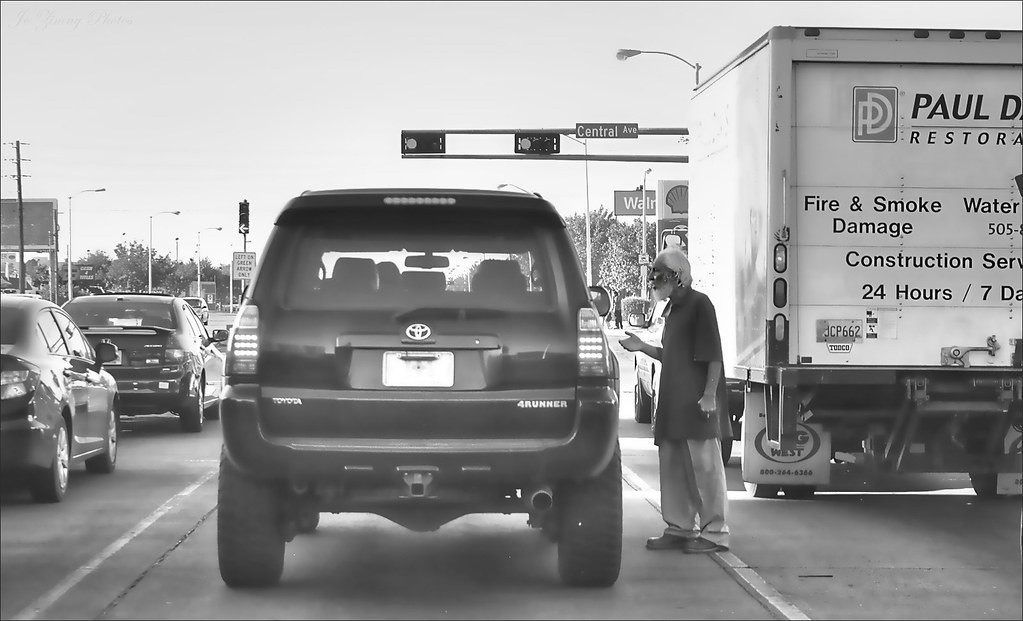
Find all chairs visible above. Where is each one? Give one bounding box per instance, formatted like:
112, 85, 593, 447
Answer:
332, 257, 379, 291
470, 260, 528, 293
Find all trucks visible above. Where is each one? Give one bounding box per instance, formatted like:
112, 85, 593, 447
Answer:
672, 26, 1020, 501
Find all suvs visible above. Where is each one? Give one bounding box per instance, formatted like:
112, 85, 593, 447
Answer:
215, 182, 630, 584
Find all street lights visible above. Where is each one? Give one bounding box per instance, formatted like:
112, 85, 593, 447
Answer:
196, 227, 222, 300
618, 45, 701, 86
149, 210, 180, 293
637, 167, 653, 298
67, 187, 106, 298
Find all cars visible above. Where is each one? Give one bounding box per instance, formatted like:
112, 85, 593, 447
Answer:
2, 290, 120, 497
183, 297, 209, 325
222, 284, 267, 389
58, 292, 224, 433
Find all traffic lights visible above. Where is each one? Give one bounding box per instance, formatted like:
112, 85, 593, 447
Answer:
398, 132, 445, 156
239, 201, 249, 235
513, 135, 564, 155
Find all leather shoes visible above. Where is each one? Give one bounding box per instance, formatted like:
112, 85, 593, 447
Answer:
647, 534, 695, 548
684, 537, 728, 553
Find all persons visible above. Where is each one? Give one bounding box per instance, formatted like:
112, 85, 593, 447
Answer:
613, 291, 624, 330
618, 248, 731, 554
